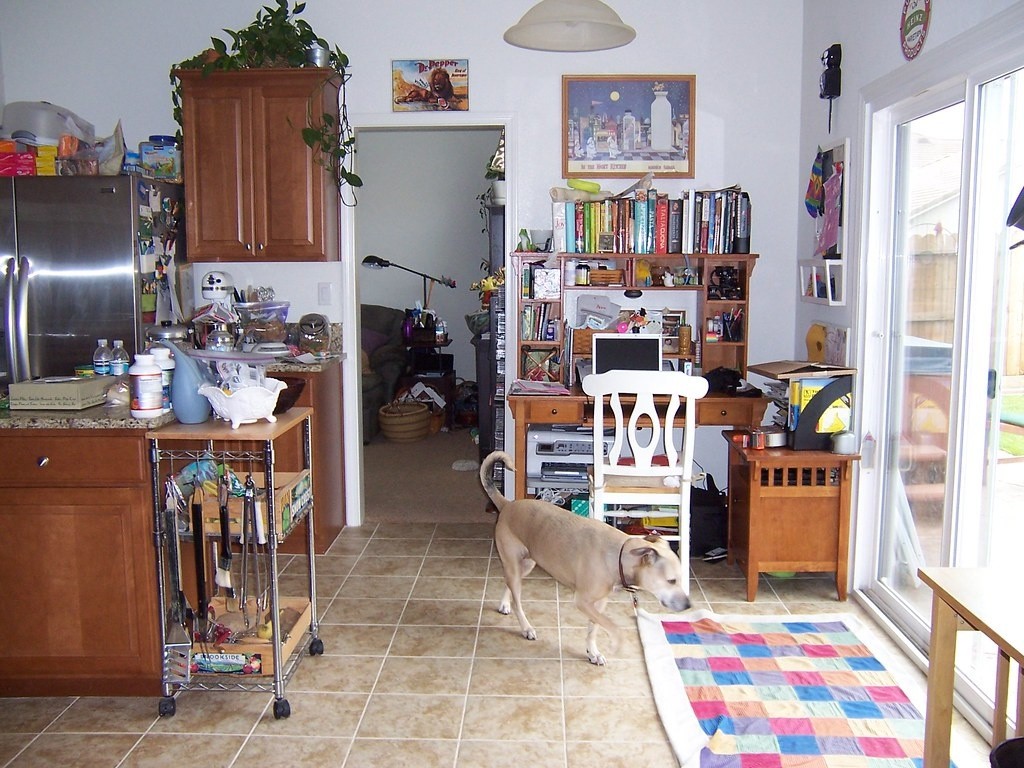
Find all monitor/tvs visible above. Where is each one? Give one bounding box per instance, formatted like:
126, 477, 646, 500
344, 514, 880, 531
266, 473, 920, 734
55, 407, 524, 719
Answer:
593, 332, 662, 376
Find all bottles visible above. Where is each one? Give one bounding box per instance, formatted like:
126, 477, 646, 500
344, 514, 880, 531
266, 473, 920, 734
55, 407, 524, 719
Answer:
150, 348, 175, 411
205, 323, 234, 352
565, 261, 575, 286
128, 354, 164, 418
575, 261, 590, 287
94, 337, 113, 376
109, 340, 129, 378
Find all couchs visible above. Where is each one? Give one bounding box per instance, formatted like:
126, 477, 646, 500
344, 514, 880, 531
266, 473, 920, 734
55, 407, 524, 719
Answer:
360, 300, 407, 441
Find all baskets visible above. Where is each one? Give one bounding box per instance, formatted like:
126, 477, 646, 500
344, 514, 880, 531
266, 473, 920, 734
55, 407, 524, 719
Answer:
431, 408, 446, 435
574, 329, 616, 354
590, 269, 624, 284
379, 388, 431, 442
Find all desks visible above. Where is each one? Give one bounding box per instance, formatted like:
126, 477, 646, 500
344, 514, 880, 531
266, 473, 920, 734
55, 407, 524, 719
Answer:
507, 388, 766, 575
708, 432, 865, 601
918, 559, 1023, 766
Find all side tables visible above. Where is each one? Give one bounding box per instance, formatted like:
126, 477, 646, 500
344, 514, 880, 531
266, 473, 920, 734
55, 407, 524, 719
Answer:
401, 336, 455, 427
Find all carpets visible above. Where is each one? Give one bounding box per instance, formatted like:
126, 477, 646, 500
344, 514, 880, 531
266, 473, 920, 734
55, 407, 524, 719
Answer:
632, 608, 957, 767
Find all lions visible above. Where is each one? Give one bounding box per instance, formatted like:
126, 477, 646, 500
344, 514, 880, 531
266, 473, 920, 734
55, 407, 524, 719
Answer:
394, 67, 464, 111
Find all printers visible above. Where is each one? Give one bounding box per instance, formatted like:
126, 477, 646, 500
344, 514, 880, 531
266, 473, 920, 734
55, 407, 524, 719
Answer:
526, 431, 616, 484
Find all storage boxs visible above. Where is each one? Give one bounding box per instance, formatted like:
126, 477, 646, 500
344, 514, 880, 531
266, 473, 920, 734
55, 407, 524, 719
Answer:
747, 358, 855, 380
194, 597, 309, 678
191, 467, 309, 538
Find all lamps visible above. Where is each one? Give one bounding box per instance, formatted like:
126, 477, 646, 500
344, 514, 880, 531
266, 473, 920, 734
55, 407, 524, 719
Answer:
361, 254, 457, 328
504, 0, 636, 54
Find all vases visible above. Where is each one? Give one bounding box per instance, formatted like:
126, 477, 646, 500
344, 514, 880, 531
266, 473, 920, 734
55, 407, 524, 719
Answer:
379, 402, 431, 443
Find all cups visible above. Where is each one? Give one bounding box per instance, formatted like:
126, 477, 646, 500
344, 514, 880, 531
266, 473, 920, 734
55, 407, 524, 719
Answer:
680, 326, 691, 354
651, 265, 670, 287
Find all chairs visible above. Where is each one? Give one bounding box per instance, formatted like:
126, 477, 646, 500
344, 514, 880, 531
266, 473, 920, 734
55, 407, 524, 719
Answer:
579, 368, 708, 594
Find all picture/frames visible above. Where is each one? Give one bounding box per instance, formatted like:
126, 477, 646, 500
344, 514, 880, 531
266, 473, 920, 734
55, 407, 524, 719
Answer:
561, 73, 700, 179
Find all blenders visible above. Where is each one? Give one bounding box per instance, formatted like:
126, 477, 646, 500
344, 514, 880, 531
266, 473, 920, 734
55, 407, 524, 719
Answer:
200, 272, 235, 311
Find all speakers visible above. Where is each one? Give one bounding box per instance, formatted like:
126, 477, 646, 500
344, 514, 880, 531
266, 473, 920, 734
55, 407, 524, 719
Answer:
662, 360, 675, 372
575, 362, 593, 386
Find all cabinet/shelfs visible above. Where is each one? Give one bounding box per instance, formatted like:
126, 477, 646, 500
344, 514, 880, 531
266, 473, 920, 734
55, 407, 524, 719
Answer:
148, 406, 320, 720
216, 362, 346, 559
515, 245, 762, 385
0, 424, 208, 695
178, 72, 343, 261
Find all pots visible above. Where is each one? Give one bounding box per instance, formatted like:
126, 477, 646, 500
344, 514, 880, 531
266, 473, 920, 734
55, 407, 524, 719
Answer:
144, 320, 189, 342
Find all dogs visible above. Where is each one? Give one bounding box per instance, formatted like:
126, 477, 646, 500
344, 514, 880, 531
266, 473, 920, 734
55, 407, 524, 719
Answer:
479, 450, 692, 669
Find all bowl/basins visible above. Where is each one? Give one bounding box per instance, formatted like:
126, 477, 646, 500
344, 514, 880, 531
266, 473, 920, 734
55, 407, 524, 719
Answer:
238, 300, 290, 324
197, 377, 288, 429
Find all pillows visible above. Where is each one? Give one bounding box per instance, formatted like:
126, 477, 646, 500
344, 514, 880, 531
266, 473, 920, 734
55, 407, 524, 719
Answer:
361, 324, 391, 357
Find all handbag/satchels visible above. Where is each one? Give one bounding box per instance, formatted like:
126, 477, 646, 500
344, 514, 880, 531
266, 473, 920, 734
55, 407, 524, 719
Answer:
690, 474, 728, 555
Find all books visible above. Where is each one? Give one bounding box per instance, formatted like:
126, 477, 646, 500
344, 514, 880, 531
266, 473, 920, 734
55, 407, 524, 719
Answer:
521, 262, 561, 300
520, 302, 561, 341
551, 186, 751, 255
747, 359, 857, 434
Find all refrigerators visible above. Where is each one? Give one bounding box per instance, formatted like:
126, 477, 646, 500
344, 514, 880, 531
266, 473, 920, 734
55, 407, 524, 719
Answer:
0, 175, 185, 407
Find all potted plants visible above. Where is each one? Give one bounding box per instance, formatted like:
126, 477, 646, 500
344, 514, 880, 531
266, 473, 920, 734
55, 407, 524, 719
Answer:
175, 0, 364, 207
479, 161, 505, 209
